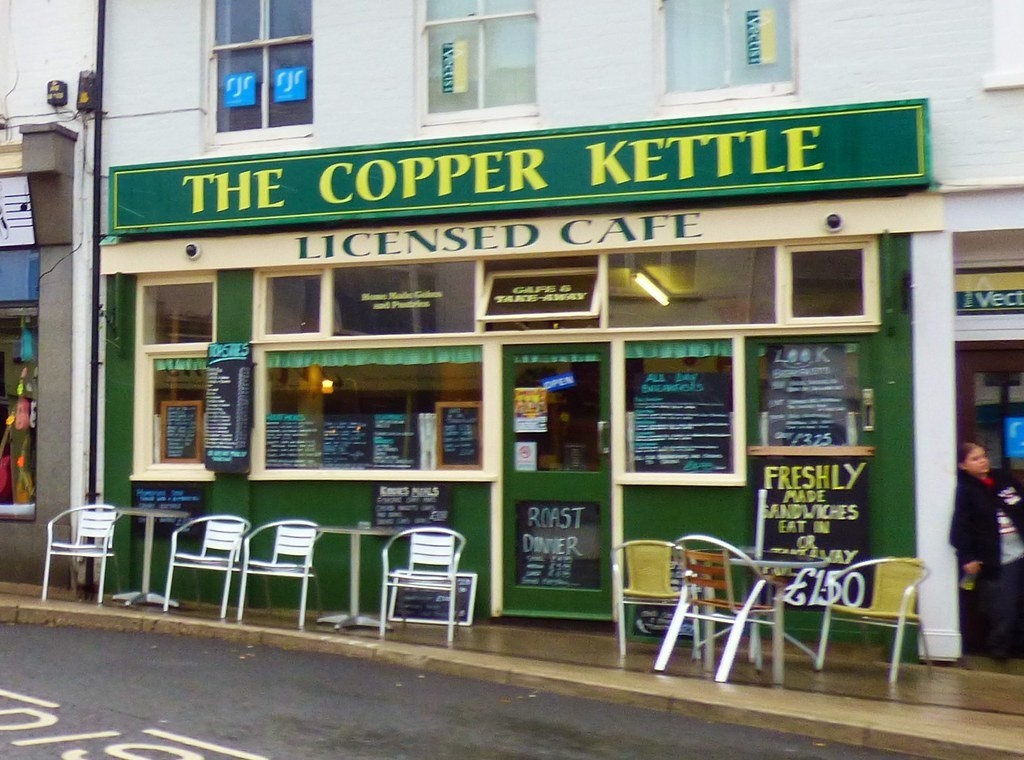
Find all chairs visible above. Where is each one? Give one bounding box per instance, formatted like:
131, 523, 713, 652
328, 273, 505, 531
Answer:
653, 535, 787, 681
813, 557, 933, 684
379, 525, 467, 643
237, 519, 324, 628
611, 539, 702, 660
163, 514, 250, 618
41, 504, 124, 603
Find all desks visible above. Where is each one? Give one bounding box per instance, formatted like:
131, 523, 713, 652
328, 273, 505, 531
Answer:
700, 551, 825, 671
317, 525, 403, 632
111, 507, 189, 606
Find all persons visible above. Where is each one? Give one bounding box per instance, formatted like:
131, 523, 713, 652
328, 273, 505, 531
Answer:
949, 442, 1024, 661
